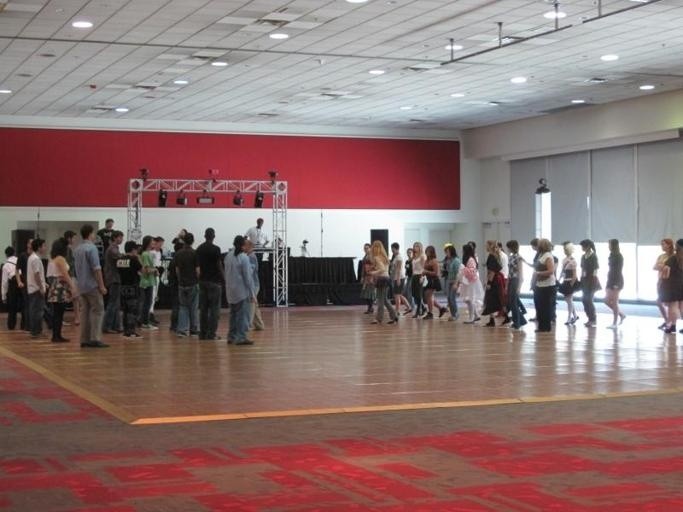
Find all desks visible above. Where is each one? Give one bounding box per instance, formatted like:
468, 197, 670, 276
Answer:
155, 256, 356, 309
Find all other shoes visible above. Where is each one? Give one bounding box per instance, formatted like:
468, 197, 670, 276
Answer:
360, 304, 627, 332
664, 325, 676, 332
658, 323, 665, 329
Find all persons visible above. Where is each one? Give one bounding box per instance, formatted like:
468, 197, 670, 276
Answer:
662, 239, 683, 333
580, 240, 603, 327
653, 239, 676, 329
559, 241, 582, 325
604, 239, 626, 328
361, 239, 558, 332
2, 218, 284, 348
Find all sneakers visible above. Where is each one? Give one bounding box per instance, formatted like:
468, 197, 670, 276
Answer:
7, 313, 268, 348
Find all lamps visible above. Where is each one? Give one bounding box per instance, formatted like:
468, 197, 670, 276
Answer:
176, 191, 187, 206
233, 191, 243, 205
255, 191, 264, 208
159, 190, 167, 207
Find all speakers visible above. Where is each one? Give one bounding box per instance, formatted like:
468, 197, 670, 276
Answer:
371, 230, 388, 256
11, 229, 35, 258
